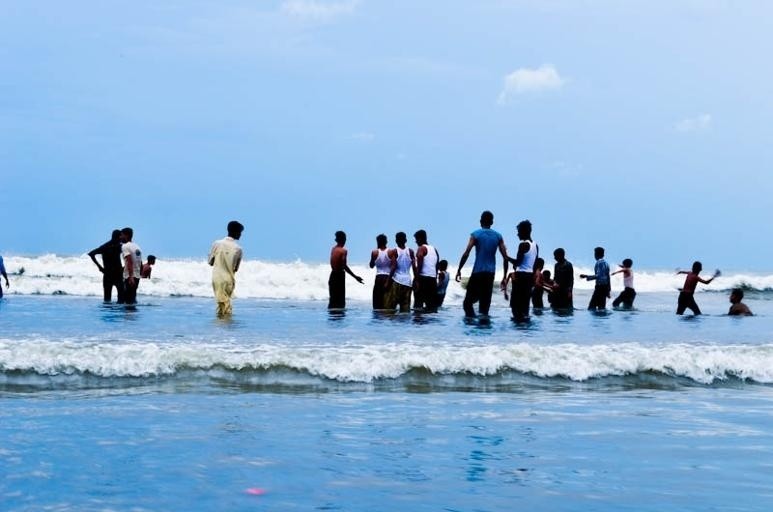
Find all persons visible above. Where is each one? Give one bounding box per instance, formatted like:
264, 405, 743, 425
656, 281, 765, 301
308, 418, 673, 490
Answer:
456, 209, 508, 317
0, 256, 10, 299
676, 262, 721, 314
328, 231, 364, 309
88, 228, 156, 310
370, 230, 450, 314
502, 220, 574, 316
728, 288, 750, 316
208, 220, 244, 314
580, 248, 611, 310
612, 260, 636, 308
